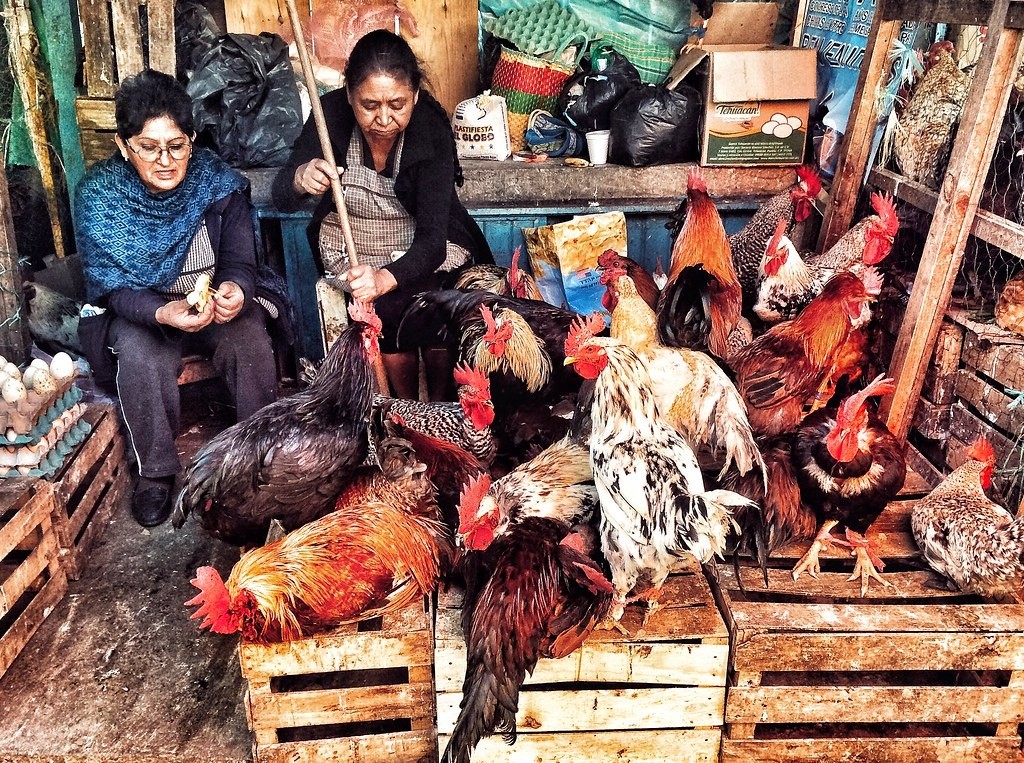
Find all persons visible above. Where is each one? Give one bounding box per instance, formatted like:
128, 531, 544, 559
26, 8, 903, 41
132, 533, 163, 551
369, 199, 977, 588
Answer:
275, 30, 497, 402
74, 67, 275, 527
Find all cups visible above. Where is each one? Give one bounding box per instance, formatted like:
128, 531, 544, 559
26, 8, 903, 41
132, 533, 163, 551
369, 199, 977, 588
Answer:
585, 131, 610, 165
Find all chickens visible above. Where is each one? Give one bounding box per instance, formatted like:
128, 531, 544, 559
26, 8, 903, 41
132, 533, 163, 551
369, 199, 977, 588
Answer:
169, 40, 1024, 763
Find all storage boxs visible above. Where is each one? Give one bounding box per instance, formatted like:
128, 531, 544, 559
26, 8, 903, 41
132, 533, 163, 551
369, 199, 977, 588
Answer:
664, 44, 818, 167
0, 276, 1024, 763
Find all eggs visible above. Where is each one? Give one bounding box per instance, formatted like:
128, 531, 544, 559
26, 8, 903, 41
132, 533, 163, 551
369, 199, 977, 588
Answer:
0, 352, 85, 475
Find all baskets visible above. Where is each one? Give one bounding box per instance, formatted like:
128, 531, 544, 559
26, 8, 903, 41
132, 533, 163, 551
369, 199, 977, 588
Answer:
492, 31, 588, 154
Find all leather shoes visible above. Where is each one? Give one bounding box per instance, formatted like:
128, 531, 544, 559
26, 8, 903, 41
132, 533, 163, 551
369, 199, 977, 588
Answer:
131, 471, 176, 527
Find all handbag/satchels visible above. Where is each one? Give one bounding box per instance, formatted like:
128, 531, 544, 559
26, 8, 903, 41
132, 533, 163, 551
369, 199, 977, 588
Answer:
523, 108, 577, 158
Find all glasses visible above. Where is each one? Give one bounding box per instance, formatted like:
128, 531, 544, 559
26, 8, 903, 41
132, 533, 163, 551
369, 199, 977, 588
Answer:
123, 137, 194, 162
